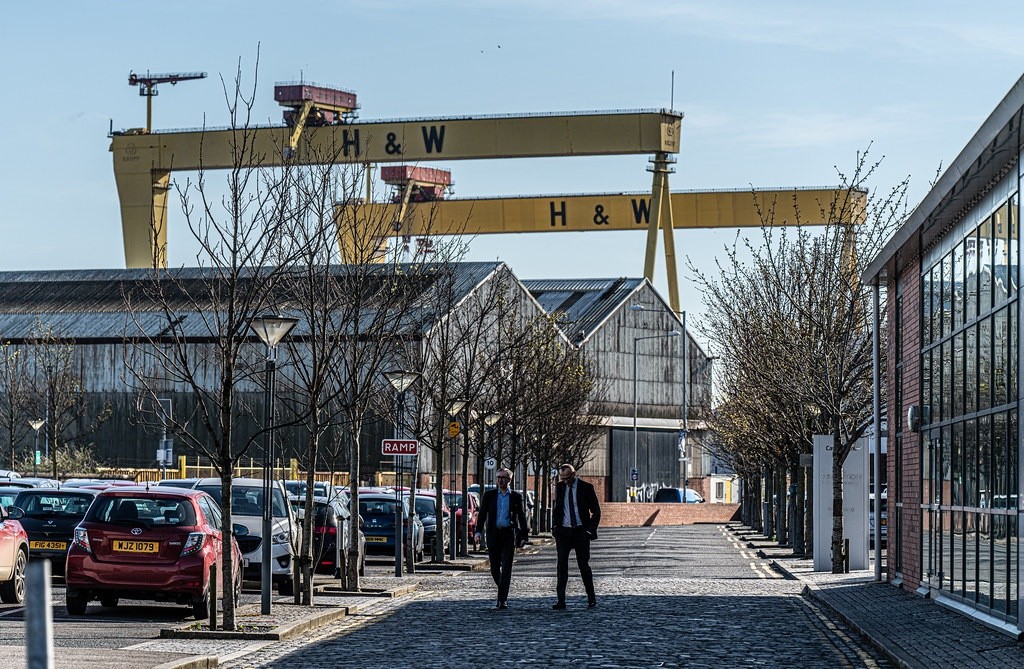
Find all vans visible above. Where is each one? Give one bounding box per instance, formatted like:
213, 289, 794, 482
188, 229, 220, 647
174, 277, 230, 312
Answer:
654, 488, 706, 503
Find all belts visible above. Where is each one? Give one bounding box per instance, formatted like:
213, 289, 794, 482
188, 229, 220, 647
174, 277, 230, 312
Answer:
496, 526, 511, 531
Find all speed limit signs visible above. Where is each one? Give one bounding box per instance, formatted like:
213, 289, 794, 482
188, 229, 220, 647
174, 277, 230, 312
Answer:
485, 458, 496, 469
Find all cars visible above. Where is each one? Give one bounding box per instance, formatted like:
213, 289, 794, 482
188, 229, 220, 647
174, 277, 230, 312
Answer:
980, 494, 1018, 533
0, 470, 534, 603
66, 485, 249, 619
869, 492, 888, 547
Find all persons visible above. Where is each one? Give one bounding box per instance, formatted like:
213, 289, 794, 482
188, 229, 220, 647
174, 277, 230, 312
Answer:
551, 464, 601, 610
473, 468, 528, 609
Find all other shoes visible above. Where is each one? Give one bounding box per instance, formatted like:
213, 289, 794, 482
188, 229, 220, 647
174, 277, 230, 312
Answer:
589, 599, 596, 607
553, 601, 566, 609
496, 598, 499, 607
499, 600, 508, 609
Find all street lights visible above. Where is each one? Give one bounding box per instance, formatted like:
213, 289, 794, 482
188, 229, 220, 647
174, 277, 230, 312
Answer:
478, 410, 504, 532
632, 332, 681, 502
243, 316, 301, 615
380, 368, 421, 579
434, 398, 470, 561
627, 305, 688, 503
28, 417, 45, 477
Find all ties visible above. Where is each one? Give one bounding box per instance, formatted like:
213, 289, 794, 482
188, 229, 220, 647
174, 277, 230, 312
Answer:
568, 486, 577, 528
